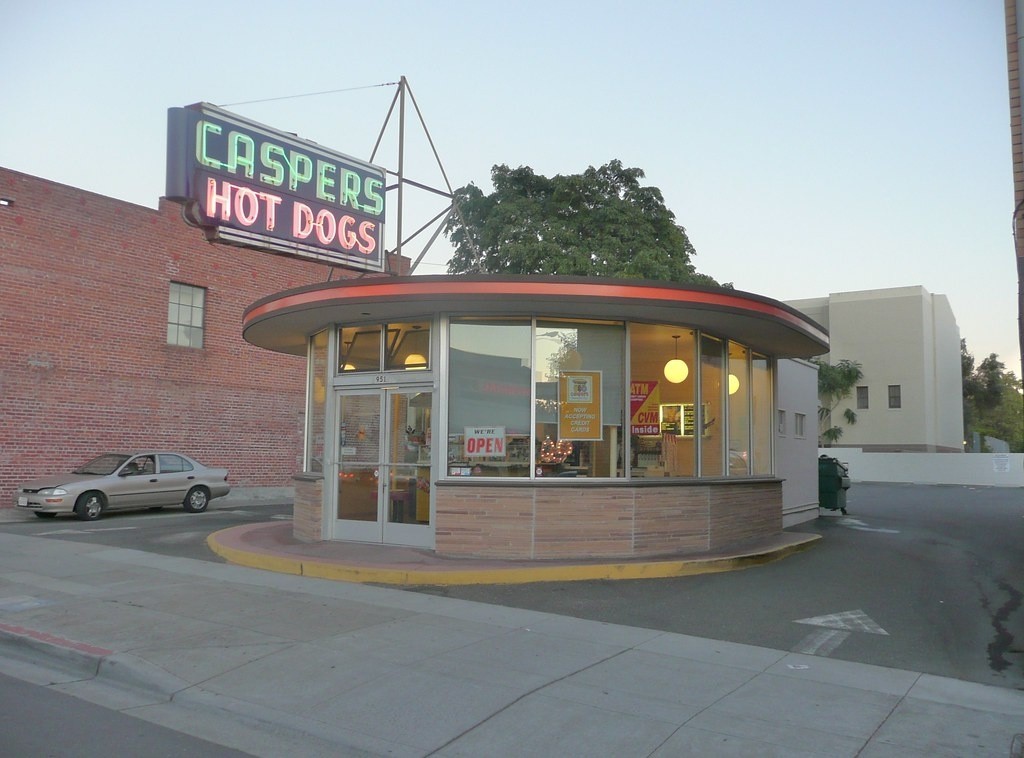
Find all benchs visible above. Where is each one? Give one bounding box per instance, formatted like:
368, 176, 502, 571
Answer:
370, 489, 412, 523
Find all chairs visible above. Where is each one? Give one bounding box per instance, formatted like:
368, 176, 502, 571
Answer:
115, 460, 140, 476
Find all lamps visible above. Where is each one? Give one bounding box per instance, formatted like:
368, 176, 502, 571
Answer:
405, 325, 426, 371
339, 341, 355, 370
716, 352, 740, 395
664, 335, 689, 384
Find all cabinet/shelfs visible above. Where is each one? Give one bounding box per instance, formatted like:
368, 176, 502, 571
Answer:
415, 434, 587, 523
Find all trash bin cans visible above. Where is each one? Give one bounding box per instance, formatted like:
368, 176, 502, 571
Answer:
818, 457, 853, 517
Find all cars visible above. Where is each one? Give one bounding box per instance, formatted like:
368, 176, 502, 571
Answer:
12, 452, 230, 521
339, 466, 372, 484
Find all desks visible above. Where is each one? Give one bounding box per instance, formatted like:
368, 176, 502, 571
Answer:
375, 474, 416, 520
